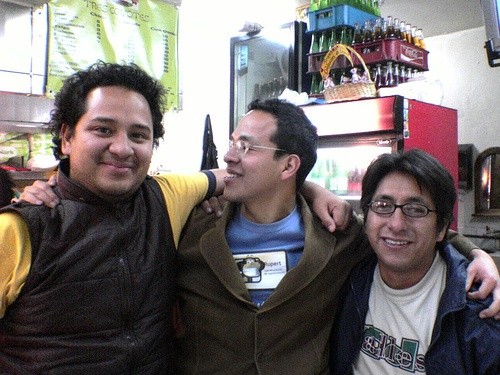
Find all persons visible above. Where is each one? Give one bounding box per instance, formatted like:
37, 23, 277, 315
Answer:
11, 98, 500, 375
201, 148, 500, 375
0, 63, 353, 375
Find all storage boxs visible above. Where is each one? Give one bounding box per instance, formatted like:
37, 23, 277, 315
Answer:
304, 5, 431, 72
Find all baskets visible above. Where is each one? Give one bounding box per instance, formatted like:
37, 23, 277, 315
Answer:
322, 46, 376, 103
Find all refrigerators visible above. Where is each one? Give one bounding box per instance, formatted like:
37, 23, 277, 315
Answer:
229, 20, 312, 145
298, 94, 459, 231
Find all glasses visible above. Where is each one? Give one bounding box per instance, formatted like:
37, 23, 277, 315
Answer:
225, 138, 290, 156
368, 199, 441, 218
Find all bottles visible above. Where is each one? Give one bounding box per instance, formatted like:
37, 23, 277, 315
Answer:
318, 30, 329, 52
308, 32, 319, 54
338, 26, 354, 47
309, 65, 376, 94
253, 83, 259, 101
268, 80, 273, 98
393, 18, 401, 40
278, 76, 285, 96
406, 24, 412, 45
382, 20, 387, 39
412, 26, 419, 47
328, 29, 339, 50
374, 61, 427, 91
387, 16, 395, 39
260, 83, 268, 102
363, 20, 376, 42
309, 0, 380, 19
418, 29, 426, 50
375, 19, 382, 39
401, 21, 406, 41
272, 78, 278, 97
354, 22, 364, 44
